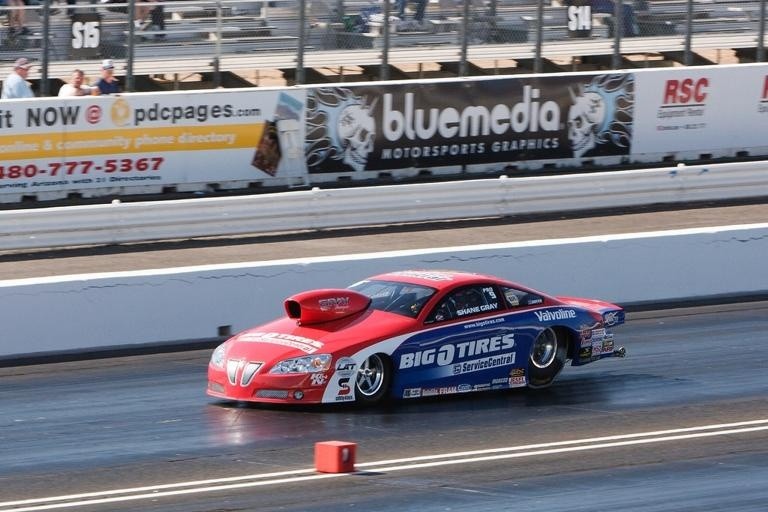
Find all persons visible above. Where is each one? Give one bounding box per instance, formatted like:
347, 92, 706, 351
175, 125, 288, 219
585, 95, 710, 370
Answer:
396, 0, 428, 25
58, 70, 101, 98
0, 57, 35, 99
90, 63, 122, 95
140, 0, 164, 32
6, 0, 25, 29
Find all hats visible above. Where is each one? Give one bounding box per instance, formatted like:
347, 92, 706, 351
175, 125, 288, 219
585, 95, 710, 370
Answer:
15, 58, 32, 70
102, 60, 114, 70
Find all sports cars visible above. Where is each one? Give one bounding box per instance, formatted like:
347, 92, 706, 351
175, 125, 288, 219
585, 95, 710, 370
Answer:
208, 271, 626, 404
